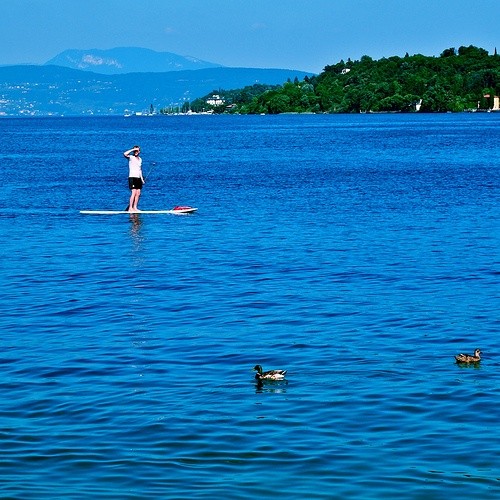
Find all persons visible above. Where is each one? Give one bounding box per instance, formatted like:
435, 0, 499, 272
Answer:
123, 145, 145, 213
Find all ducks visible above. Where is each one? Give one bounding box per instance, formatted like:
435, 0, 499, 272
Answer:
250, 364, 288, 381
454, 348, 482, 362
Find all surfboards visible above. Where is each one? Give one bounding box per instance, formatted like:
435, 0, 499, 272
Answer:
78, 205, 198, 214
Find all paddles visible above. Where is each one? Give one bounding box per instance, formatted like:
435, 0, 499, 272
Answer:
134, 162, 156, 212
